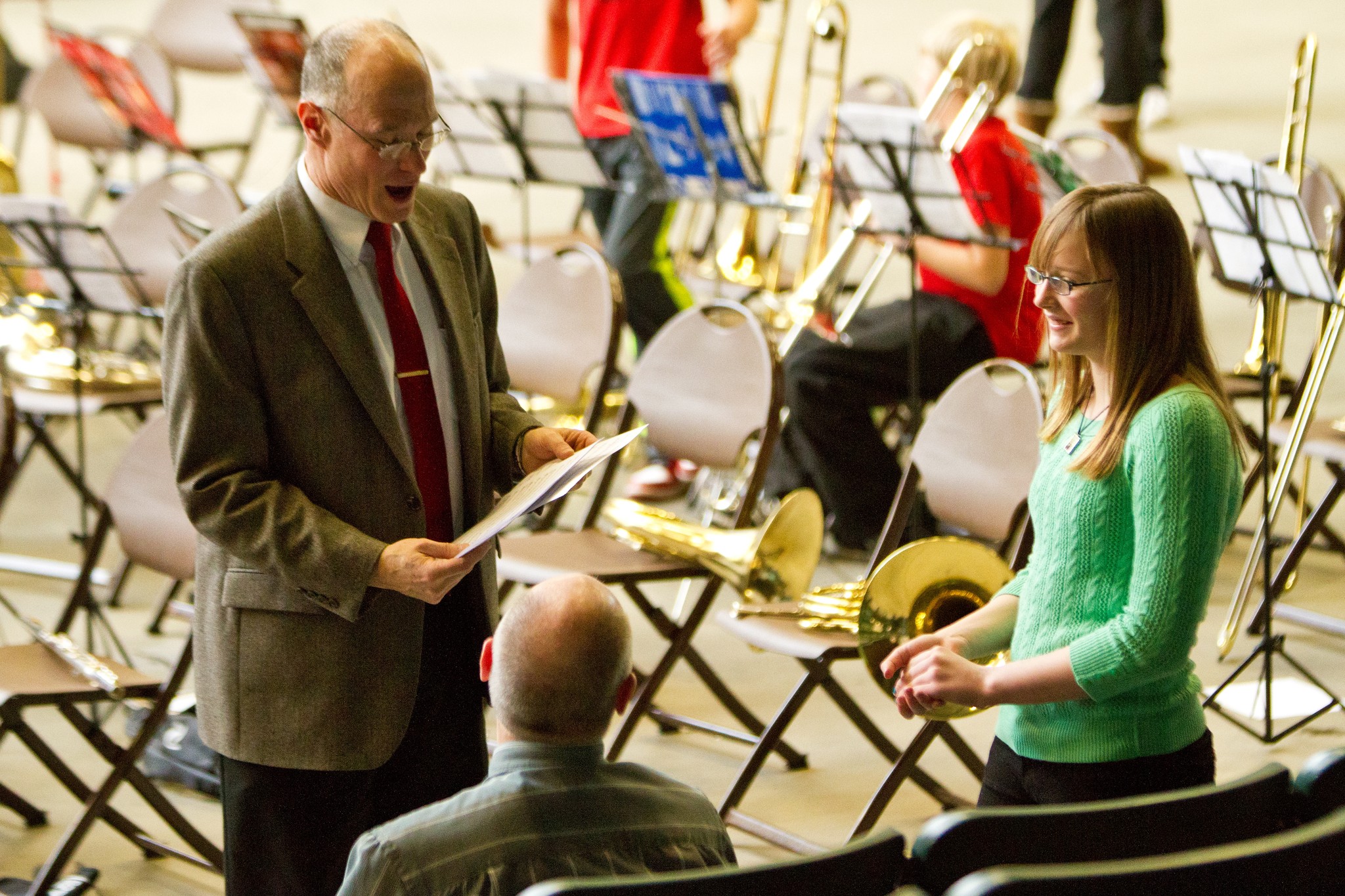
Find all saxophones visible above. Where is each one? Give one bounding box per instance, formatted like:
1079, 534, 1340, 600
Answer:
734, 533, 1014, 720
599, 490, 825, 609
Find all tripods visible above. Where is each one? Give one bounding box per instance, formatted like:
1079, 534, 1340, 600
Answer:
0, 198, 163, 726
1173, 147, 1345, 742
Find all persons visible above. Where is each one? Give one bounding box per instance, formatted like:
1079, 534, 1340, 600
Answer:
781, 20, 1048, 553
160, 18, 597, 896
882, 182, 1245, 813
1014, 0, 1169, 176
576, 0, 759, 352
335, 573, 737, 896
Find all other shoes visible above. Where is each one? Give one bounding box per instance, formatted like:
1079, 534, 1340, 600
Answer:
711, 495, 778, 530
821, 526, 907, 560
625, 458, 699, 498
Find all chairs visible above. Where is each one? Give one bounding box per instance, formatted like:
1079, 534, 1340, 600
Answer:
0, 0, 1345, 896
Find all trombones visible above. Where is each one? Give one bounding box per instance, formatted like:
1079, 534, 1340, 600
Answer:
694, 32, 1012, 525
1211, 273, 1344, 666
1236, 32, 1321, 594
715, 0, 850, 292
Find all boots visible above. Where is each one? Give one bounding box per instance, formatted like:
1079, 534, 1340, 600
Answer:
1015, 97, 1055, 139
1097, 103, 1169, 177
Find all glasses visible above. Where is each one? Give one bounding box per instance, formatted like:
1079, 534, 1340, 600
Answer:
1024, 265, 1113, 296
319, 107, 452, 161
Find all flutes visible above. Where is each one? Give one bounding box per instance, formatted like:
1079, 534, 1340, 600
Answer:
38, 632, 125, 701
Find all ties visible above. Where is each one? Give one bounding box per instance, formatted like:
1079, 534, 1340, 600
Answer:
365, 220, 455, 544
